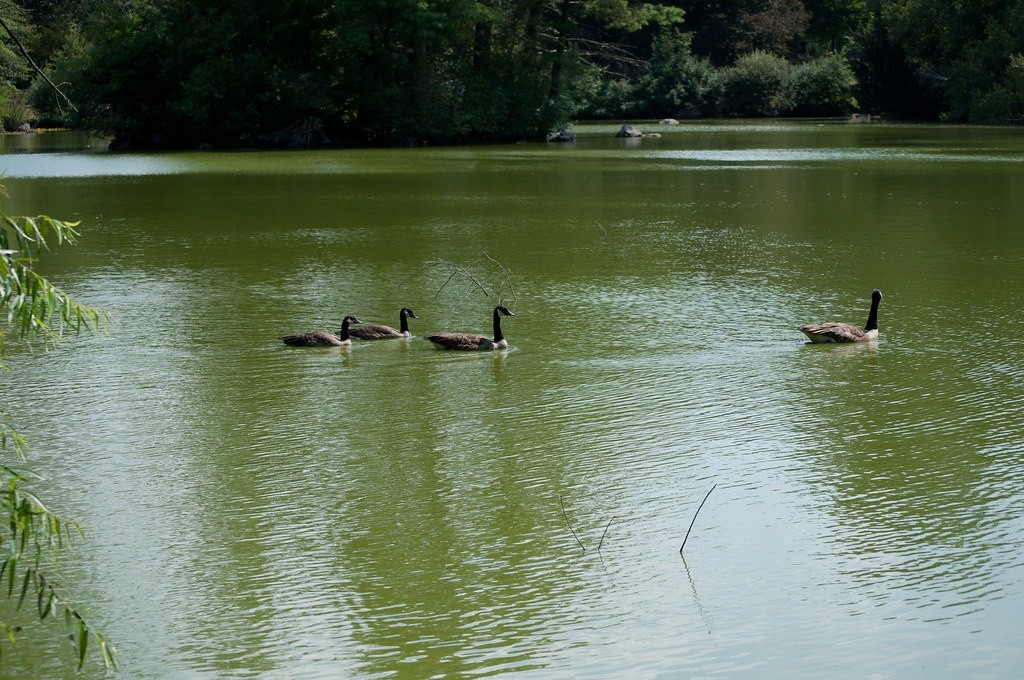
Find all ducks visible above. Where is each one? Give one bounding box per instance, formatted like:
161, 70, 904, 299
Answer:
424, 306, 516, 350
799, 290, 882, 343
277, 307, 419, 346
